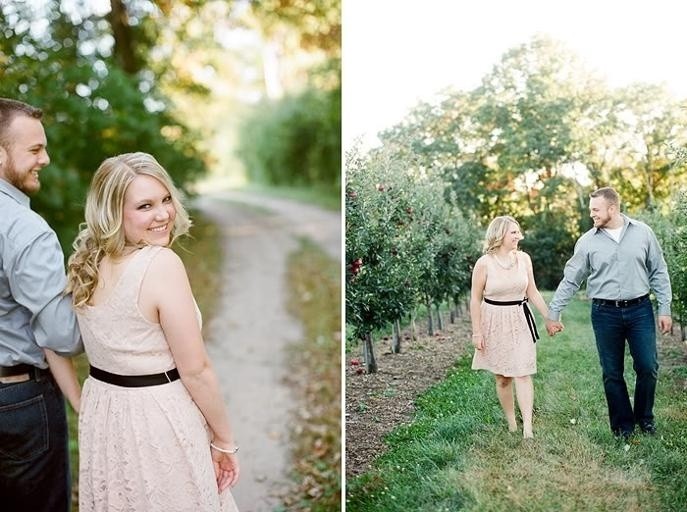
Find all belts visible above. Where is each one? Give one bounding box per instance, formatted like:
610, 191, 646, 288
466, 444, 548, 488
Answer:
593, 294, 650, 308
0, 365, 50, 387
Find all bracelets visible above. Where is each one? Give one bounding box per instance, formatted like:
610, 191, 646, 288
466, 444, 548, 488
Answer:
471, 335, 483, 338
210, 441, 239, 454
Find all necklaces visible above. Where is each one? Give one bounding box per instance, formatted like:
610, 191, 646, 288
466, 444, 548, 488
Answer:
492, 251, 515, 271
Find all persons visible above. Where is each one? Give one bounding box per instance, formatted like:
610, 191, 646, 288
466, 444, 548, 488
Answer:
468, 215, 564, 438
44, 150, 240, 512
543, 187, 673, 446
0, 95, 85, 512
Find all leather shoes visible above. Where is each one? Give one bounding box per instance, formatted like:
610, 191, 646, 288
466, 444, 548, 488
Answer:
615, 422, 656, 439
